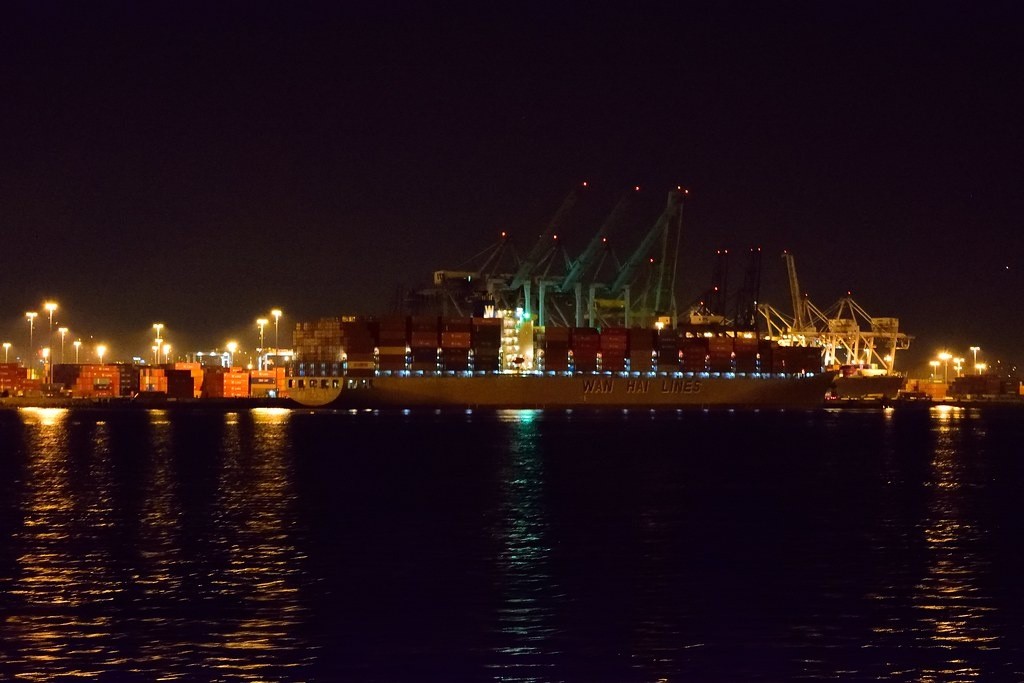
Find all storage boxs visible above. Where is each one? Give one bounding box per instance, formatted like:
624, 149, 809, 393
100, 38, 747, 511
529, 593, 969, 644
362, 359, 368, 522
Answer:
0, 297, 1023, 397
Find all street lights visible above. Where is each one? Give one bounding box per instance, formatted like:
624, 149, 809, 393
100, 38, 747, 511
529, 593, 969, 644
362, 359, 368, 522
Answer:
929, 359, 939, 398
952, 346, 991, 381
270, 309, 282, 385
254, 318, 269, 367
2, 343, 12, 364
57, 327, 69, 365
25, 311, 38, 381
44, 301, 59, 395
149, 323, 172, 366
226, 340, 238, 369
73, 341, 82, 363
95, 343, 108, 364
938, 351, 952, 389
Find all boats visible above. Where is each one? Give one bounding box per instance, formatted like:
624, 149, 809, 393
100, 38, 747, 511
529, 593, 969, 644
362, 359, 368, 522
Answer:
280, 177, 843, 418
888, 390, 942, 413
